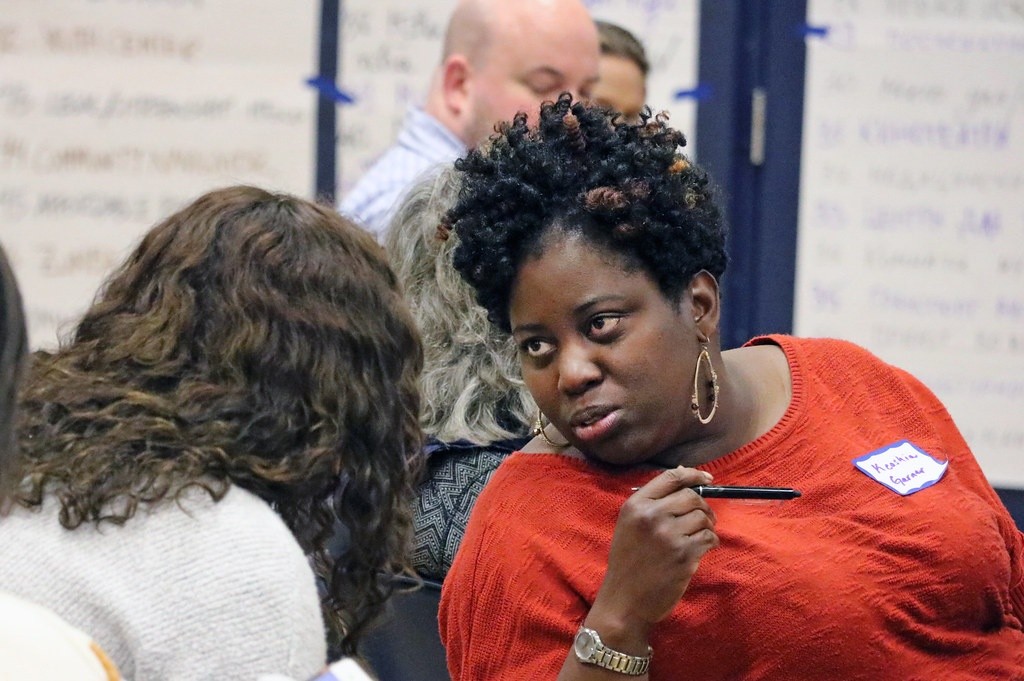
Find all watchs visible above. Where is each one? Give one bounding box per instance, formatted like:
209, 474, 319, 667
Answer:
574, 626, 653, 675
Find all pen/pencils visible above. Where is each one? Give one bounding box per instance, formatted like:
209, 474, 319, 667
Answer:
631, 486, 802, 500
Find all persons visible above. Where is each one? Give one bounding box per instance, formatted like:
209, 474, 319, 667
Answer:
437, 97, 1024, 681
0, 186, 423, 681
590, 20, 648, 118
0, 245, 124, 681
335, 0, 599, 252
382, 164, 554, 582
